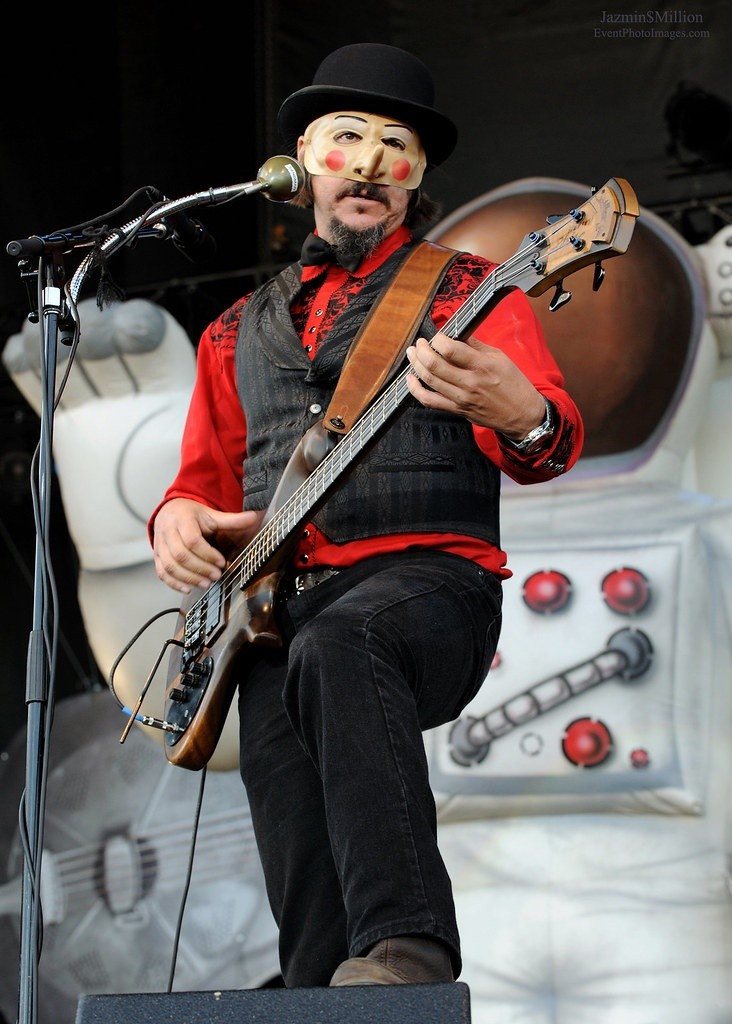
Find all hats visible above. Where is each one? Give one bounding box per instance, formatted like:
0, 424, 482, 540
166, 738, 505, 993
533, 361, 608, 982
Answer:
278, 42, 457, 173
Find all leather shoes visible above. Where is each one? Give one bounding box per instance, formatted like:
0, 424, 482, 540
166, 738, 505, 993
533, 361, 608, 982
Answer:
328, 932, 454, 987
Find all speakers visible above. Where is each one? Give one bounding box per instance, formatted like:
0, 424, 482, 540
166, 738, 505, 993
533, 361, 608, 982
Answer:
75, 981, 472, 1024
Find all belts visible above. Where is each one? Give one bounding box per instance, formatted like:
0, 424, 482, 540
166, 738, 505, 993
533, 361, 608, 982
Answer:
283, 566, 341, 601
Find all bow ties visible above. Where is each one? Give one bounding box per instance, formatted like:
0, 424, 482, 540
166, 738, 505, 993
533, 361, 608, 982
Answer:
300, 232, 362, 273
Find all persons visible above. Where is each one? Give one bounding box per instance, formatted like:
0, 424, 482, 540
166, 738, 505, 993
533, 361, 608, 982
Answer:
147, 42, 582, 985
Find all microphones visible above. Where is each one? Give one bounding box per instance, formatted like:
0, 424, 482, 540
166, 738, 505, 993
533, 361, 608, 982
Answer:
146, 186, 205, 249
245, 156, 306, 204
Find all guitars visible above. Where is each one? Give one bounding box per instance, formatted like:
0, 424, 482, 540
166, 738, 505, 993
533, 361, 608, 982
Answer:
162, 170, 639, 773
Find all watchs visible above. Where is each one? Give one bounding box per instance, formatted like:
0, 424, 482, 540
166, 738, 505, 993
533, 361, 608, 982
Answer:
508, 400, 557, 455
